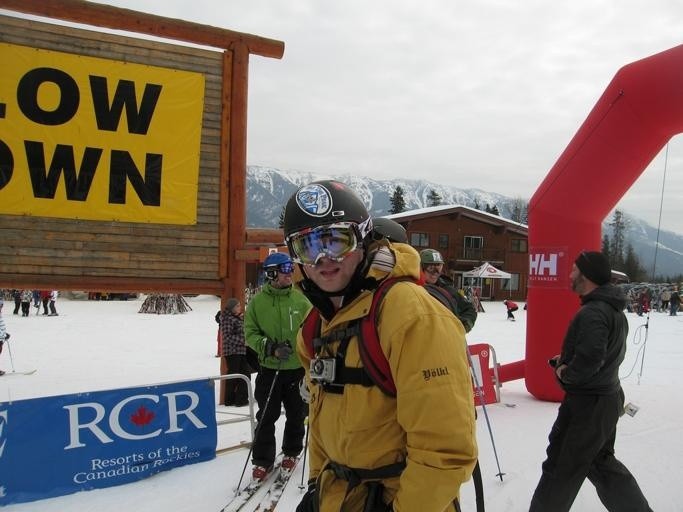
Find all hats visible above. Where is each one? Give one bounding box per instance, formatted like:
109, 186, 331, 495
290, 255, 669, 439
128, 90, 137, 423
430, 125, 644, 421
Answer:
575, 251, 611, 285
225, 297, 238, 310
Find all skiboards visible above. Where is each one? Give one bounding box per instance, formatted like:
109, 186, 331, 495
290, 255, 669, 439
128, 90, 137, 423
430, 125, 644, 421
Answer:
220, 440, 306, 512
5, 369, 36, 375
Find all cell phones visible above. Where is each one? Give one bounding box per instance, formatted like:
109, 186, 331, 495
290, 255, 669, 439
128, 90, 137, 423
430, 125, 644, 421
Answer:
547, 359, 558, 367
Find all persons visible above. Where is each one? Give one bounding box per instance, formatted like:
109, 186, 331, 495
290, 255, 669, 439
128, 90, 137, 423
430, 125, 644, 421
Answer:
529, 252, 653, 512
0, 288, 59, 375
219, 298, 253, 406
285, 180, 478, 512
599, 281, 683, 316
243, 252, 313, 482
503, 298, 518, 321
419, 248, 477, 334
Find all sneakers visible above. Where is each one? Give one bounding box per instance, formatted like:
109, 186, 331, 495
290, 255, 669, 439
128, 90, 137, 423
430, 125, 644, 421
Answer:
224, 400, 250, 407
282, 455, 296, 468
253, 466, 267, 478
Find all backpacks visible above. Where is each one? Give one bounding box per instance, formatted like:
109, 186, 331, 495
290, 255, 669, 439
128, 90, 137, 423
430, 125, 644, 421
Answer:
301, 275, 457, 481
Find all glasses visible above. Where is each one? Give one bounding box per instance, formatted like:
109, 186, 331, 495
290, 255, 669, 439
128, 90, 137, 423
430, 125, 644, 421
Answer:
266, 263, 295, 274
292, 215, 373, 268
423, 264, 443, 274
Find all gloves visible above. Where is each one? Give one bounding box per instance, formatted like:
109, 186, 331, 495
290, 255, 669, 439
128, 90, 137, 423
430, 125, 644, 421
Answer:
270, 342, 292, 359
548, 355, 564, 372
296, 477, 319, 512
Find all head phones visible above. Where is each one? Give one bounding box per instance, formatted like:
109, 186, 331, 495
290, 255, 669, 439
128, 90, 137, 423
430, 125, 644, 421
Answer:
265, 267, 278, 280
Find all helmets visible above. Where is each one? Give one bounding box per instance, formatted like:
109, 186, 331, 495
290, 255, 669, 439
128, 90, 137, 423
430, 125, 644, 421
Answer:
284, 180, 409, 243
263, 252, 293, 280
420, 249, 445, 264
